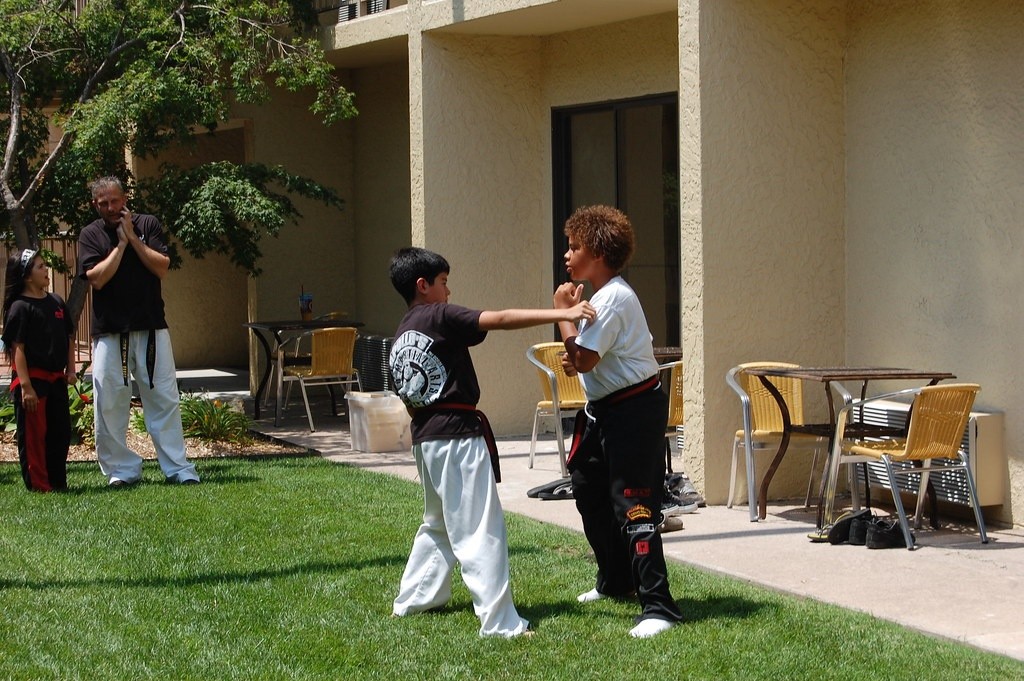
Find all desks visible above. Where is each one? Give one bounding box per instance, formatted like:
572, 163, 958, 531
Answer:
746, 365, 959, 533
242, 319, 366, 427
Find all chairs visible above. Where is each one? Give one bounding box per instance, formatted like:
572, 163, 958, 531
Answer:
264, 312, 351, 420
843, 382, 990, 551
281, 326, 364, 432
726, 360, 860, 523
659, 360, 683, 472
526, 341, 587, 479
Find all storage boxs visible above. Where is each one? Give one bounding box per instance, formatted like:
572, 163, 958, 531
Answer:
344, 390, 413, 452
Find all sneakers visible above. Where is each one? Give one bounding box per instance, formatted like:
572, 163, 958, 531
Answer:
848, 515, 884, 544
659, 495, 680, 516
666, 471, 705, 506
667, 476, 684, 497
108, 476, 126, 486
866, 520, 916, 549
176, 471, 201, 485
664, 485, 698, 512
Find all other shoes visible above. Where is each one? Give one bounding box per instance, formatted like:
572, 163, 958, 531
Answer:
656, 513, 684, 532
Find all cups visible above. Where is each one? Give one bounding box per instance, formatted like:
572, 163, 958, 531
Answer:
299, 294, 313, 321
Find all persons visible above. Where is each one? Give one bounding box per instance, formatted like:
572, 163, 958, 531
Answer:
75, 174, 203, 491
0, 246, 77, 492
552, 203, 683, 641
385, 247, 599, 640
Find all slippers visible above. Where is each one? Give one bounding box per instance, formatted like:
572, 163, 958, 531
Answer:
538, 483, 574, 499
527, 477, 572, 497
807, 521, 836, 542
828, 508, 872, 545
